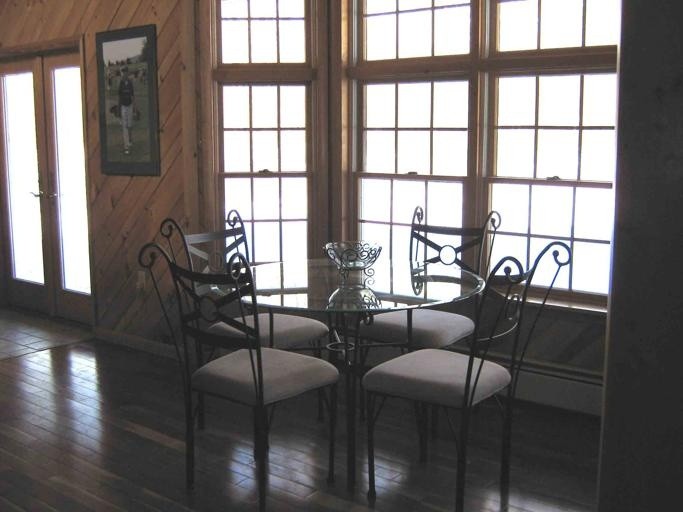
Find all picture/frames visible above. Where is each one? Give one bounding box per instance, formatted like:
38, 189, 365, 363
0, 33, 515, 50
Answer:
94, 23, 160, 177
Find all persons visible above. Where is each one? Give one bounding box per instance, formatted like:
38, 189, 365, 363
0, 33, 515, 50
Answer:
104, 66, 113, 92
114, 65, 138, 157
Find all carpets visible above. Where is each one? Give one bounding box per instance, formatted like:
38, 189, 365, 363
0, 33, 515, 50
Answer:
0, 307, 96, 361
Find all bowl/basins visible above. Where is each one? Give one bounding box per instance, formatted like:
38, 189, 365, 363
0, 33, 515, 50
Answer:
325, 241, 382, 268
327, 285, 382, 310
